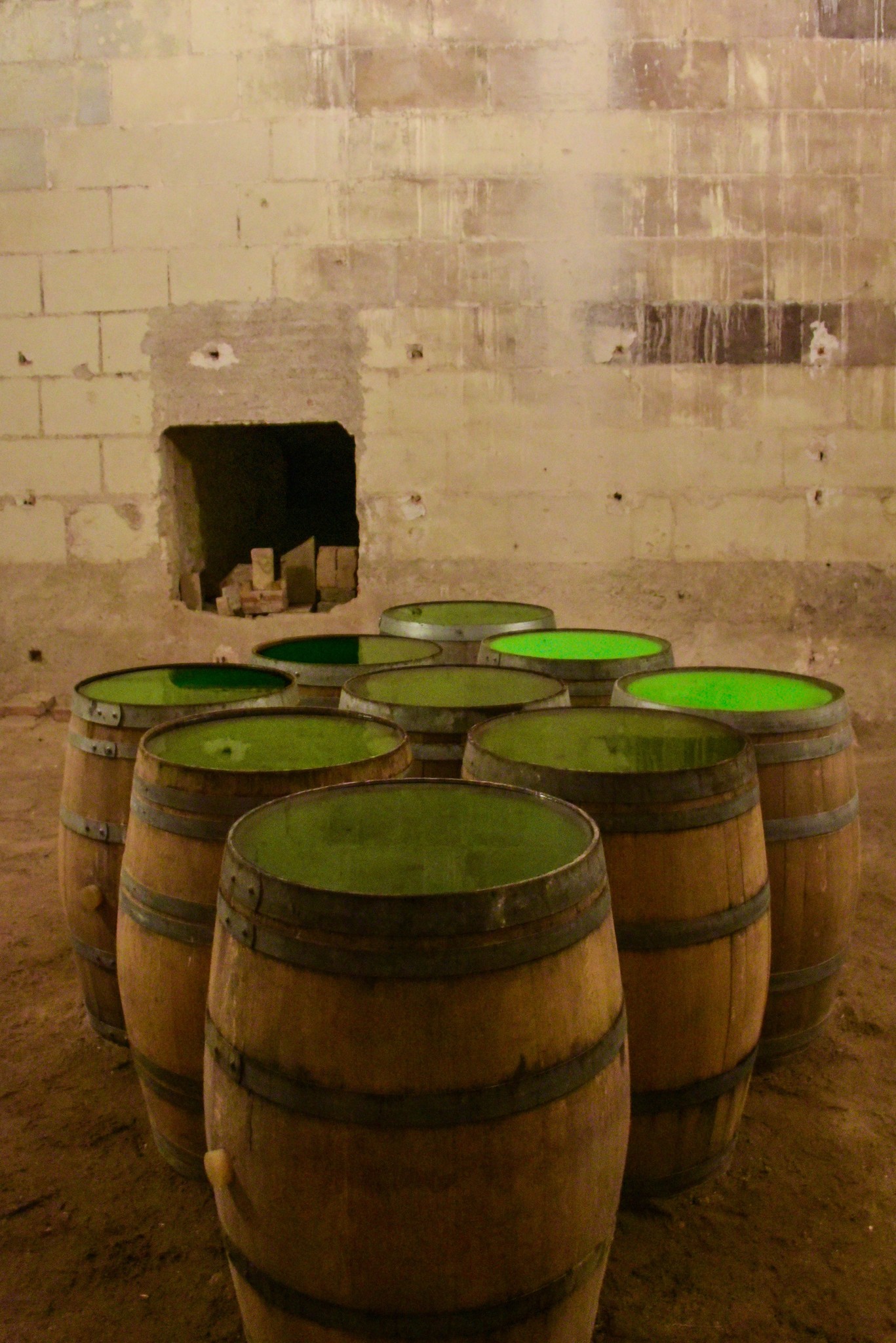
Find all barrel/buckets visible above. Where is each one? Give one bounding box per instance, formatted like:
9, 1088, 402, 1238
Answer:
613, 666, 862, 1056
376, 601, 556, 667
204, 779, 631, 1343
251, 633, 446, 709
338, 664, 571, 777
115, 708, 413, 1179
462, 706, 774, 1198
476, 626, 675, 708
59, 661, 300, 1051
